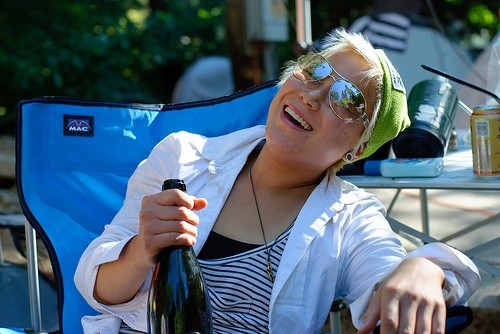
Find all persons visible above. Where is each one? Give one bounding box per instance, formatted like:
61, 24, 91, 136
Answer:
73, 28, 481, 334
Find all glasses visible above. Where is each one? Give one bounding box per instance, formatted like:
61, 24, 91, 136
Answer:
292, 46, 370, 131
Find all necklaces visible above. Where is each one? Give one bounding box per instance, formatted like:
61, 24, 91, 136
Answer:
249, 163, 297, 283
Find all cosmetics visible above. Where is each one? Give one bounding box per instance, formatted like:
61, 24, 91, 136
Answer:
364, 156, 444, 177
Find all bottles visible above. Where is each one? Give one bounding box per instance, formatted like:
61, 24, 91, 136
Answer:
392, 72, 458, 157
146, 179, 214, 334
363, 158, 444, 177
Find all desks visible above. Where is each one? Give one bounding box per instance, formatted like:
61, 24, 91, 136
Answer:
338, 129, 500, 307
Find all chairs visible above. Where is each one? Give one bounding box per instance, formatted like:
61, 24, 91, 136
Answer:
0, 213, 60, 334
14, 79, 474, 334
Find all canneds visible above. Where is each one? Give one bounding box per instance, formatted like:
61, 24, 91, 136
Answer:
469, 105, 500, 176
